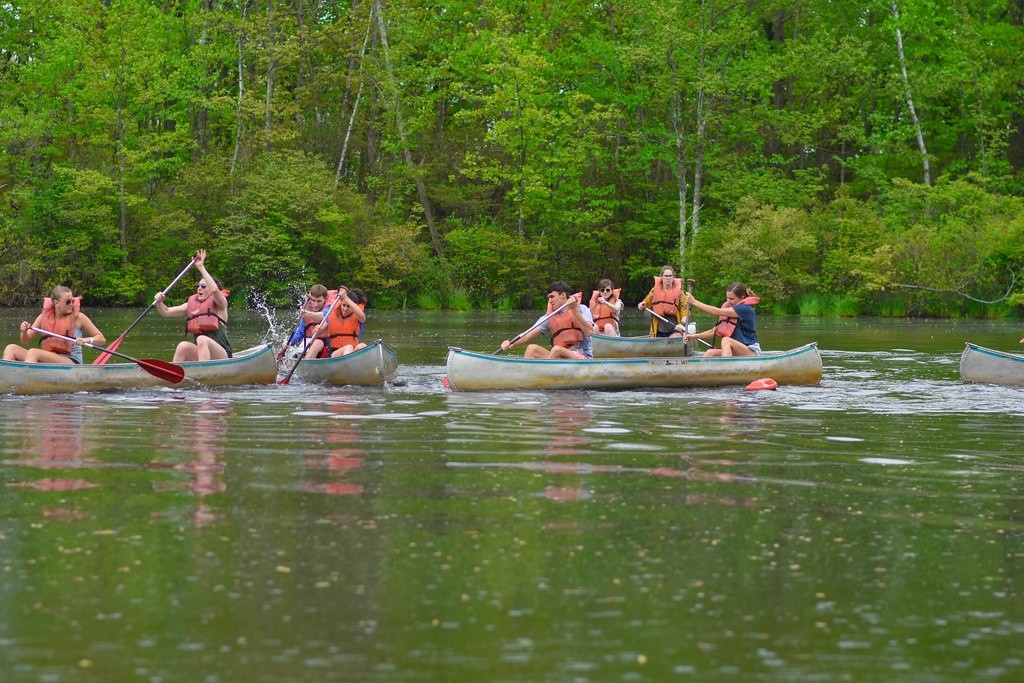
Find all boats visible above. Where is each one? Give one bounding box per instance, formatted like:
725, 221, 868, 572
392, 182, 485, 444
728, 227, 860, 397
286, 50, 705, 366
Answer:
586, 319, 702, 360
438, 341, 824, 389
0, 341, 280, 395
956, 337, 1023, 389
282, 339, 400, 386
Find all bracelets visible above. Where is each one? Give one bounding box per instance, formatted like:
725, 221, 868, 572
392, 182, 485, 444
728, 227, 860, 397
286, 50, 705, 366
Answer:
89, 336, 94, 346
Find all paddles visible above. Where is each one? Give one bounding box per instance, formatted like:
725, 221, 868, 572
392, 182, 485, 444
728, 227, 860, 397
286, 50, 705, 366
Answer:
442, 298, 573, 386
274, 285, 347, 384
27, 253, 200, 384
641, 278, 715, 356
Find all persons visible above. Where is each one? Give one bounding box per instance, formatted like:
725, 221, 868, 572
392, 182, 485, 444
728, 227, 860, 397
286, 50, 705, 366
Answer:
153, 249, 232, 363
589, 279, 625, 338
299, 288, 368, 358
2, 285, 107, 363
637, 265, 689, 337
283, 283, 337, 360
500, 281, 595, 359
682, 281, 762, 357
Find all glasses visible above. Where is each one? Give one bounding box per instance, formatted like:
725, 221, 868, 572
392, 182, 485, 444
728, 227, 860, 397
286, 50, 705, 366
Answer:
195, 283, 204, 288
600, 288, 611, 292
58, 298, 74, 305
662, 273, 673, 277
